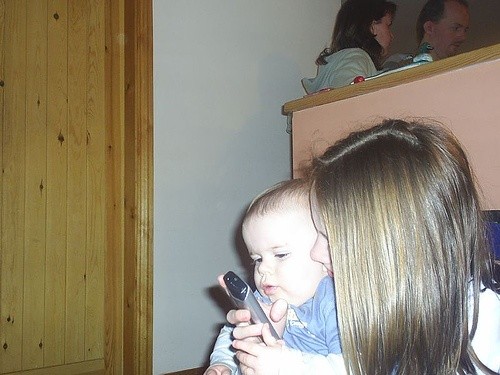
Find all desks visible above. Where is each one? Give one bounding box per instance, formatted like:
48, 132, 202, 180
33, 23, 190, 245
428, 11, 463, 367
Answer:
281, 42, 500, 294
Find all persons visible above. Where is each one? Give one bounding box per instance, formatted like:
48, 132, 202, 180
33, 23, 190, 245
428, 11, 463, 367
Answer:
226, 120, 500, 375
302, 0, 397, 94
202, 178, 361, 375
379, 0, 470, 73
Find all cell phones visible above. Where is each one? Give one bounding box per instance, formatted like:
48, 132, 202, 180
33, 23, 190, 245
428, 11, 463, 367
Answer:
224, 271, 279, 344
